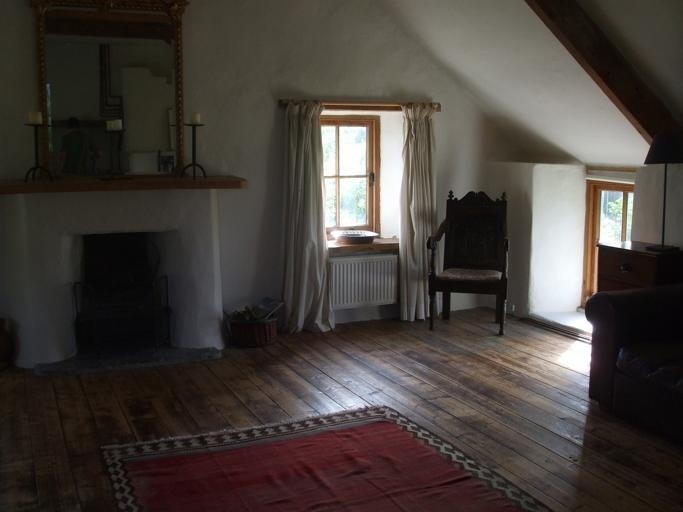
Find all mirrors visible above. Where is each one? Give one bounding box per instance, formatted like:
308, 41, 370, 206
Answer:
32, 2, 185, 180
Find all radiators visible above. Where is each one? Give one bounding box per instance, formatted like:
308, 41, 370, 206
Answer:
325, 253, 399, 311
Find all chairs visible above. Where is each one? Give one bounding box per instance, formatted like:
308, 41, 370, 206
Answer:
582, 284, 682, 449
424, 188, 513, 335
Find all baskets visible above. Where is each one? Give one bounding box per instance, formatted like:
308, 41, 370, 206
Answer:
231, 317, 277, 347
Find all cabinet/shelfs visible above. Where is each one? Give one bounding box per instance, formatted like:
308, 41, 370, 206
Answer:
595, 241, 681, 293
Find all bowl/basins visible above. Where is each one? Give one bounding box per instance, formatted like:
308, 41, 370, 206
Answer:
330, 229, 380, 246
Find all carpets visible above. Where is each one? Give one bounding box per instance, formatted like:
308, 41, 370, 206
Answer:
99, 401, 555, 511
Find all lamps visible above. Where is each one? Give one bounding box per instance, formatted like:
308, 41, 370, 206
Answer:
644, 129, 681, 253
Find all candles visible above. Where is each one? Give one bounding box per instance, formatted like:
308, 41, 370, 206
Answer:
107, 119, 122, 132
27, 111, 42, 124
189, 112, 200, 125
